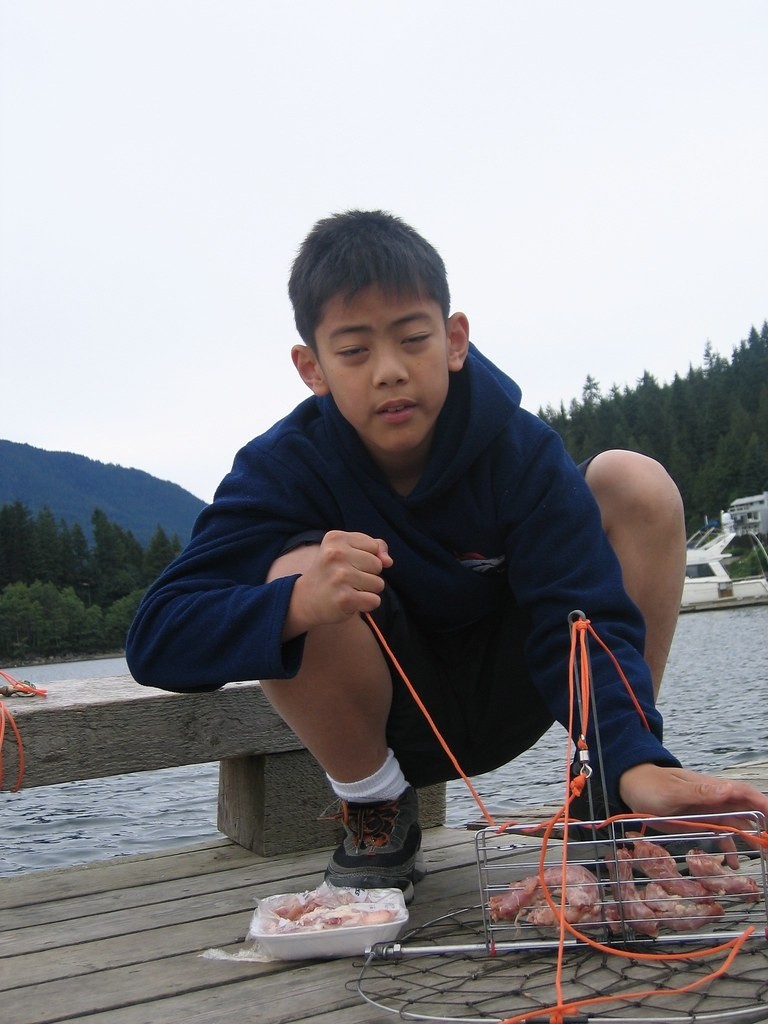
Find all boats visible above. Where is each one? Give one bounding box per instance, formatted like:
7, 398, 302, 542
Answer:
670, 514, 768, 612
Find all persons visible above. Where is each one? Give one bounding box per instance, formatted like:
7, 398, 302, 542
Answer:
125, 210, 767, 907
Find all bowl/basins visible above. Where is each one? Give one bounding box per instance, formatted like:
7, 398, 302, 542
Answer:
249, 888, 409, 961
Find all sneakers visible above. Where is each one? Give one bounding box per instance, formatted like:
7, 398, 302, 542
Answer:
325, 784, 427, 902
566, 766, 761, 887
